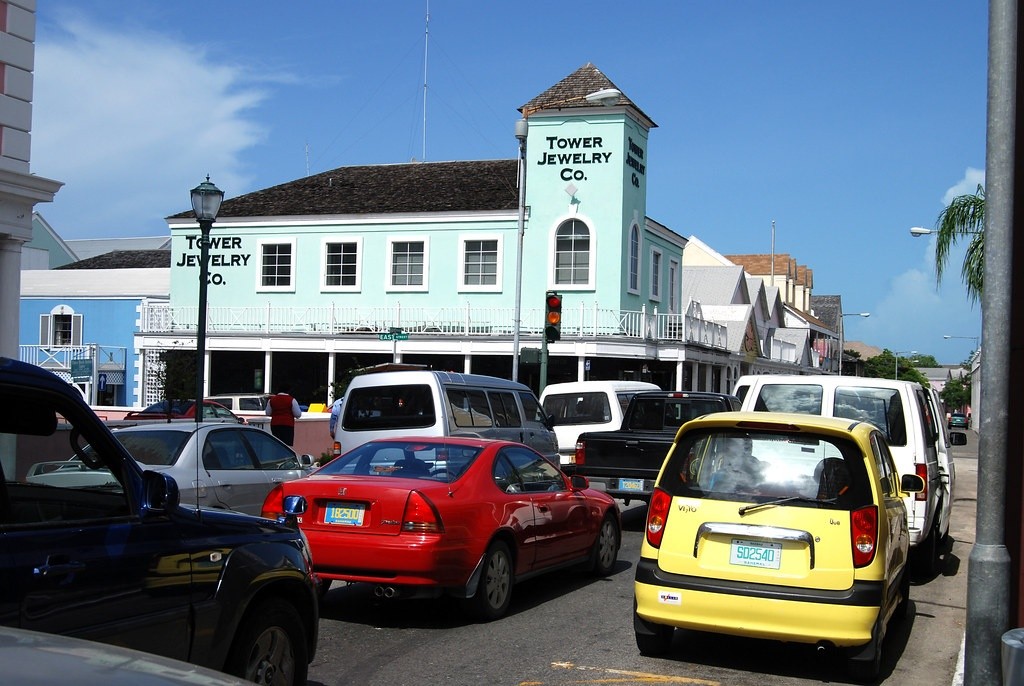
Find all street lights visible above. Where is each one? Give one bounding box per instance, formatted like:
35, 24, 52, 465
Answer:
191, 172, 226, 425
943, 335, 979, 351
896, 350, 917, 380
839, 312, 871, 375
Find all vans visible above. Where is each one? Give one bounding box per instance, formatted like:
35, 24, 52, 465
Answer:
728, 373, 969, 579
332, 371, 562, 480
535, 380, 664, 477
202, 393, 270, 425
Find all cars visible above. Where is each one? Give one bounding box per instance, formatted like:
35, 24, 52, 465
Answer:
25, 423, 322, 519
124, 398, 246, 427
948, 414, 971, 430
262, 435, 623, 625
631, 411, 928, 684
0, 623, 260, 686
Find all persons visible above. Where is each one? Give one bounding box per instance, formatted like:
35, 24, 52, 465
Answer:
265, 382, 301, 447
329, 396, 358, 439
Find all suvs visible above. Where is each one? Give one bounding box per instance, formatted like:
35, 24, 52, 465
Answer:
0, 355, 321, 686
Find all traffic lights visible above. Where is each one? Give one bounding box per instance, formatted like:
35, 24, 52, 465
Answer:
545, 294, 562, 341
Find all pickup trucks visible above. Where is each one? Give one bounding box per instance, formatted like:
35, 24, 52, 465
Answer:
569, 391, 743, 506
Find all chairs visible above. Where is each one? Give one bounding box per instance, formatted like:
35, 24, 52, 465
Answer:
390, 459, 431, 477
807, 458, 847, 500
